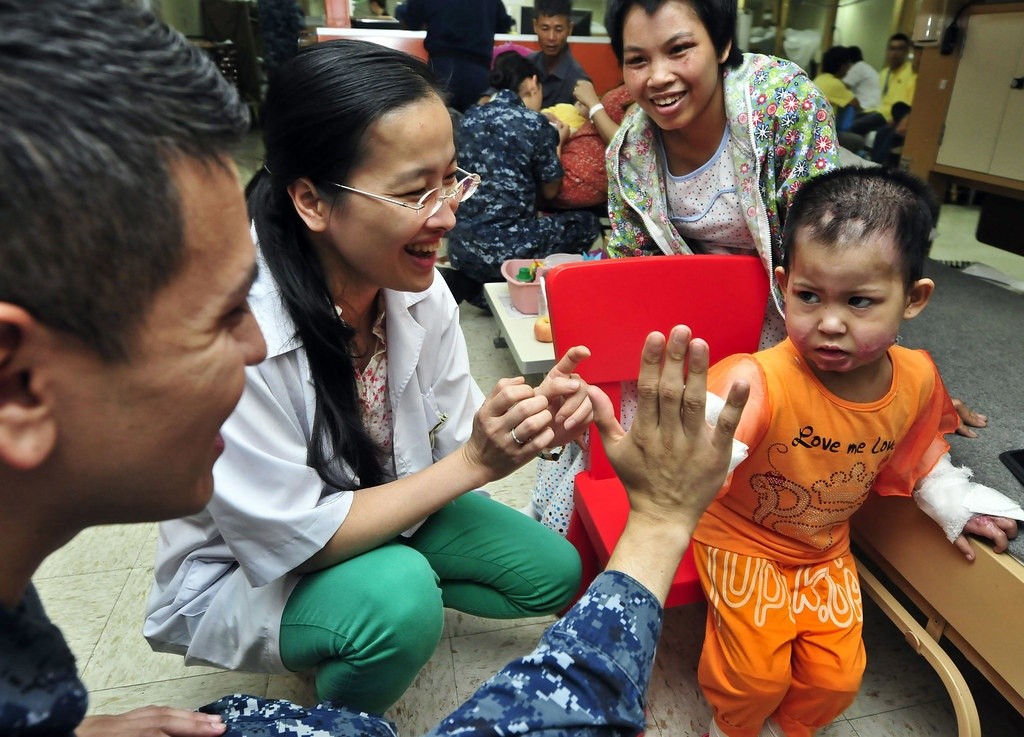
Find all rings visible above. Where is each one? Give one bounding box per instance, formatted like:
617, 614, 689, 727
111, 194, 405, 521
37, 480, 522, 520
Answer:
511, 429, 524, 444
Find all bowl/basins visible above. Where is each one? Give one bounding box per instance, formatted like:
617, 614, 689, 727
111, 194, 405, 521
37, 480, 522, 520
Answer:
501, 259, 542, 314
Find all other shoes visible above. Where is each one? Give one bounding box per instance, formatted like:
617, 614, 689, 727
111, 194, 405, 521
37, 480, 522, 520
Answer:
461, 260, 505, 313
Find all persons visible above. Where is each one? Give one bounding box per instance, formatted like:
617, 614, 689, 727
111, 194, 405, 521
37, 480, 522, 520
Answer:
846, 32, 923, 167
693, 166, 1019, 737
255, 0, 311, 87
0, 0, 751, 737
841, 46, 881, 110
812, 45, 865, 155
588, 103, 604, 124
395, 0, 515, 113
143, 37, 584, 718
519, 0, 839, 538
369, 1, 388, 16
435, 50, 599, 311
444, 0, 594, 147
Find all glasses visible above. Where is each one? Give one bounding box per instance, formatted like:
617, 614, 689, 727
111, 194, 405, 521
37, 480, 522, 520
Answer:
321, 166, 481, 219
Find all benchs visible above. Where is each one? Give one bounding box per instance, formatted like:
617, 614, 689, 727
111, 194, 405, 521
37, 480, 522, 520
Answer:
314, 28, 629, 105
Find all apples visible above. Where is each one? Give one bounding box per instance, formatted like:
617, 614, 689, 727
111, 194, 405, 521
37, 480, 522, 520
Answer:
534, 315, 553, 343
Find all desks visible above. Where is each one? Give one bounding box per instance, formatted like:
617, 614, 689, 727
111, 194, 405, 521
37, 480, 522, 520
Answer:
483, 282, 567, 374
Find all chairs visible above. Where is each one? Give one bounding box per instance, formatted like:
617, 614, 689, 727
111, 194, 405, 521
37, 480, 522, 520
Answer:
542, 255, 780, 631
